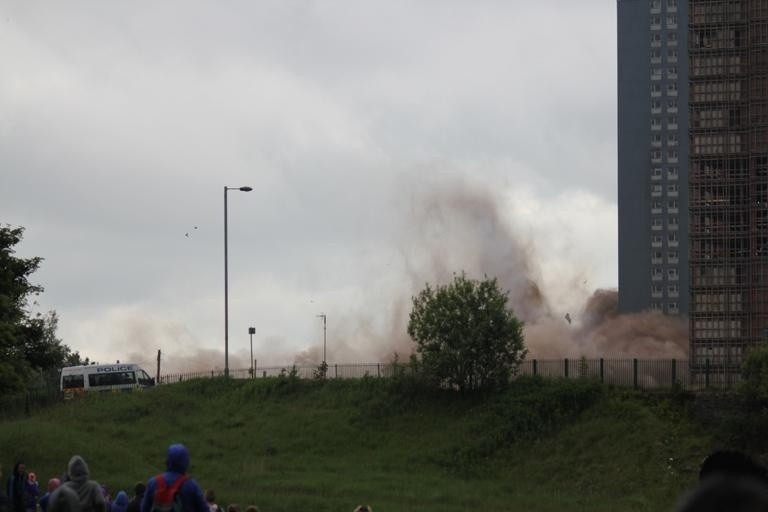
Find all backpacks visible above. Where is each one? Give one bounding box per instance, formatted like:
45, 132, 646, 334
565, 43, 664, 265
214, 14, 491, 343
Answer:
152, 475, 189, 511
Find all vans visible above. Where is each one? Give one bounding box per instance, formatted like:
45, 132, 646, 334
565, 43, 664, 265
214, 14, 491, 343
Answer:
59, 363, 154, 401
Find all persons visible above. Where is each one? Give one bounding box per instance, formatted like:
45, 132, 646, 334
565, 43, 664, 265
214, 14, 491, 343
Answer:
3, 442, 219, 512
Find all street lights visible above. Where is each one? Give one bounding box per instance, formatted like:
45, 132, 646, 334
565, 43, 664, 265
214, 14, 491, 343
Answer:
320, 315, 326, 382
224, 187, 251, 377
